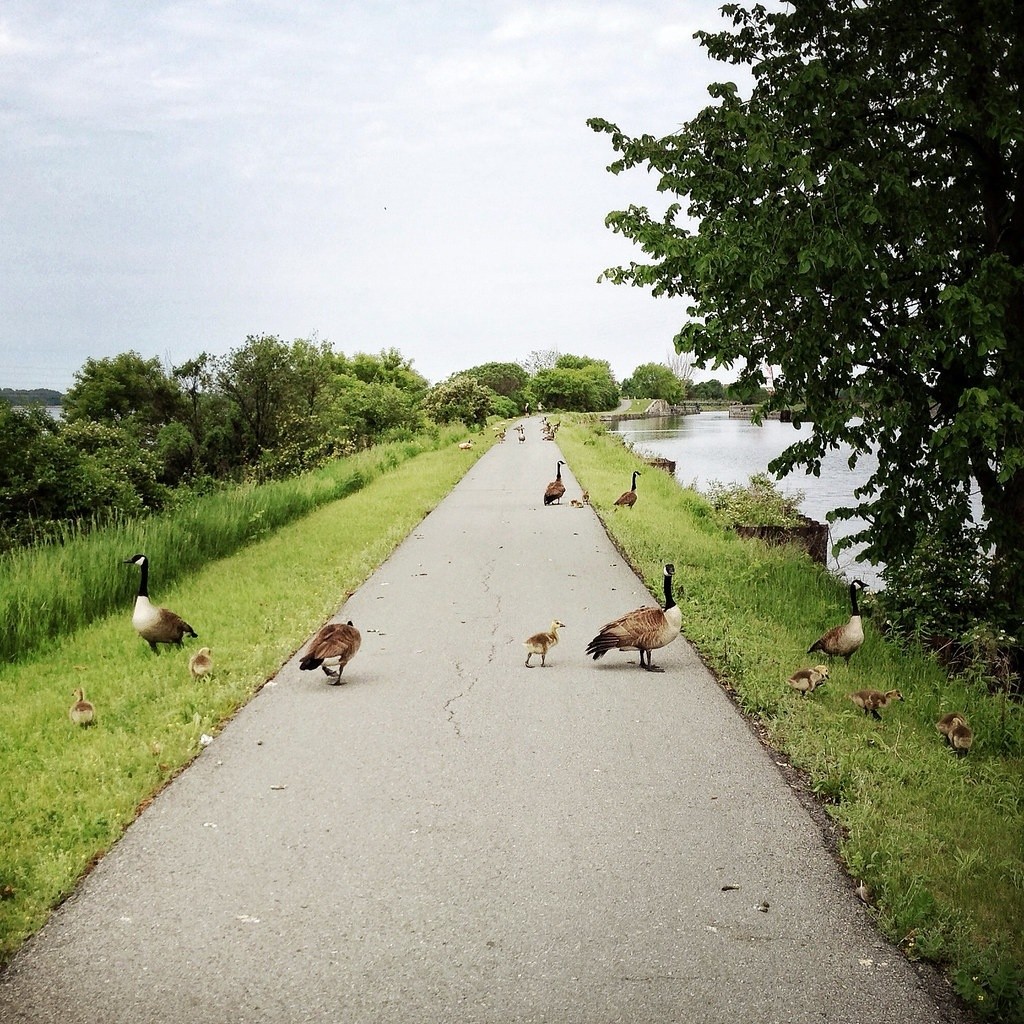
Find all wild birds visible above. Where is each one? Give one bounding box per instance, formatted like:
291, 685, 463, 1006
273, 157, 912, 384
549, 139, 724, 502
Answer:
70, 686, 95, 727
569, 490, 590, 508
543, 460, 567, 506
806, 579, 871, 666
188, 647, 216, 682
513, 424, 526, 443
612, 471, 643, 509
297, 620, 362, 686
123, 555, 198, 655
539, 415, 561, 442
935, 712, 974, 760
585, 563, 684, 673
466, 428, 507, 444
519, 620, 567, 669
786, 665, 831, 698
848, 687, 905, 722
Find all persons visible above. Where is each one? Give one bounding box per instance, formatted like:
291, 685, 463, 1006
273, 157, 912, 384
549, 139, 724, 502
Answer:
524, 402, 530, 418
536, 401, 544, 417
